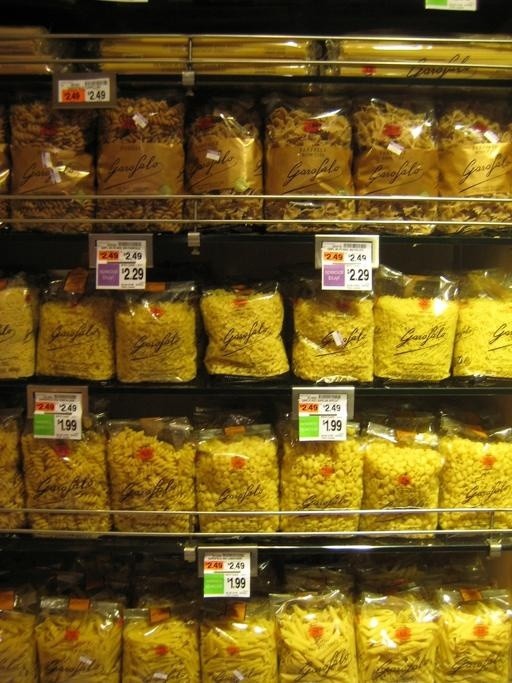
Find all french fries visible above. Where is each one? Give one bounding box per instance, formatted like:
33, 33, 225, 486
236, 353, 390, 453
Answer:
0, 552, 512, 683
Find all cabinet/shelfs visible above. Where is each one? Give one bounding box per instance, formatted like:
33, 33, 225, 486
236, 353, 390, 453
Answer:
0, 23, 511, 563
